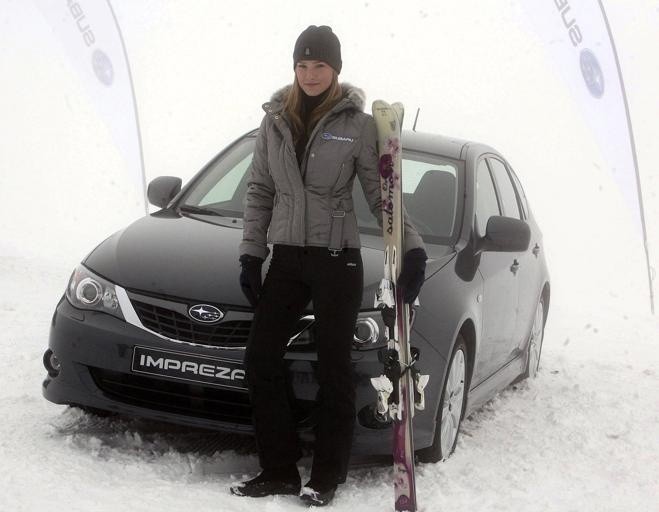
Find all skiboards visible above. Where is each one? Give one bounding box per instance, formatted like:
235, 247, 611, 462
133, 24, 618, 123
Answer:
373, 100, 415, 509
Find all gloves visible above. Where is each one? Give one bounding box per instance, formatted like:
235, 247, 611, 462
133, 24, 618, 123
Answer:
238, 253, 265, 307
396, 248, 428, 305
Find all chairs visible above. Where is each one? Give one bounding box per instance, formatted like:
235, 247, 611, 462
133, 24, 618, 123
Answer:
407, 170, 456, 240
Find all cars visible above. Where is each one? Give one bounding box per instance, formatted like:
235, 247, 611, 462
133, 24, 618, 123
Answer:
40, 119, 553, 463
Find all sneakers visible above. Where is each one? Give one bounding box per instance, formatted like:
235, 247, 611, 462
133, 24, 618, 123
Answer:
299, 478, 336, 506
229, 473, 302, 497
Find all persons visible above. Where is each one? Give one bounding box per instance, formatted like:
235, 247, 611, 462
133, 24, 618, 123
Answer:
226, 23, 431, 507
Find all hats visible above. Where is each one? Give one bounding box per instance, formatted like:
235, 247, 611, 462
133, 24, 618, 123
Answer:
293, 25, 342, 75
